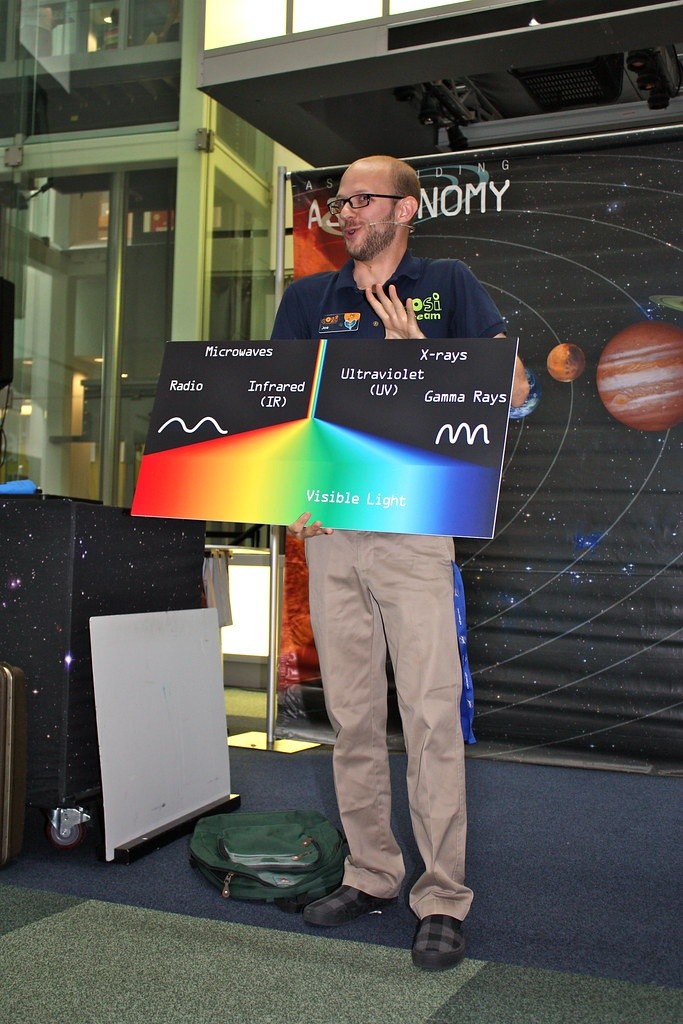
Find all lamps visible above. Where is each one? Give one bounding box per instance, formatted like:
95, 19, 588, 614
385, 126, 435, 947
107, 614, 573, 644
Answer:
418, 94, 439, 126
444, 124, 469, 152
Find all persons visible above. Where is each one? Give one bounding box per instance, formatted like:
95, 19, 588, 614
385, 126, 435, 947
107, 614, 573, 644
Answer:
272, 157, 528, 969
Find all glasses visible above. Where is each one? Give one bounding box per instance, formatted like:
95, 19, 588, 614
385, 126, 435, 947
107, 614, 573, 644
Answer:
327, 193, 405, 215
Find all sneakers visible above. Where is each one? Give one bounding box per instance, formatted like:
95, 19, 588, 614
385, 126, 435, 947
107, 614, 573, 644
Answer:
412, 914, 466, 969
303, 885, 397, 927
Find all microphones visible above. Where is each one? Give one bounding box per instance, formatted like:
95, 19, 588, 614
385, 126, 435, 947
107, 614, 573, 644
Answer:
369, 222, 415, 230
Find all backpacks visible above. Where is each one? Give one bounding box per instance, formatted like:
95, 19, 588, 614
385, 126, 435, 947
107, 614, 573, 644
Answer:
189, 809, 348, 914
